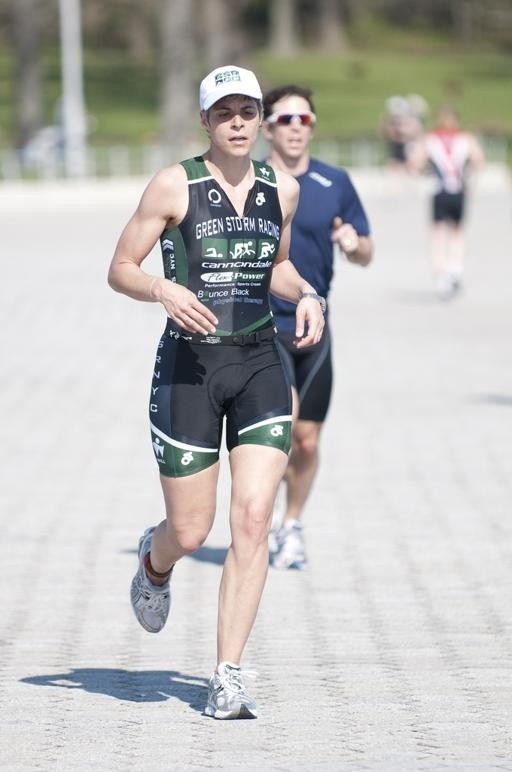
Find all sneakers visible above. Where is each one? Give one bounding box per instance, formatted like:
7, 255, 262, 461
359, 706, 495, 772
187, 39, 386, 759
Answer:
131, 527, 171, 632
205, 661, 256, 720
268, 515, 310, 570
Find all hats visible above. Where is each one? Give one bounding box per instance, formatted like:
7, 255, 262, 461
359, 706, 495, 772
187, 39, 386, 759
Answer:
199, 65, 263, 111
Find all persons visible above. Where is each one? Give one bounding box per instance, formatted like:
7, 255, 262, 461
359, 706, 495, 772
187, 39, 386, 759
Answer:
108, 63, 328, 720
261, 85, 373, 572
378, 95, 424, 174
405, 104, 488, 298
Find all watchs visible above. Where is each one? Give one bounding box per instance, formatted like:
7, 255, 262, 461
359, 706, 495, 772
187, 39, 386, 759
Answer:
300, 292, 326, 314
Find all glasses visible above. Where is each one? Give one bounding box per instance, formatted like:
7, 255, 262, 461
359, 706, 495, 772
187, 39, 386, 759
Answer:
263, 111, 317, 126
